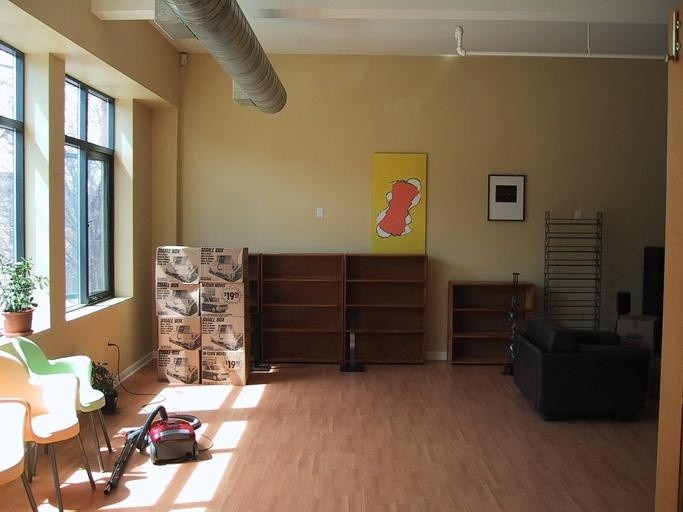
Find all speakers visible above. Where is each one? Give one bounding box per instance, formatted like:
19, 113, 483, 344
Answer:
617, 292, 630, 315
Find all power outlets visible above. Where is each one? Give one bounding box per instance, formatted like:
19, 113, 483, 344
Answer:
104, 337, 116, 351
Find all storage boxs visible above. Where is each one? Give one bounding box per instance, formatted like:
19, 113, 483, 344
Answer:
153, 245, 249, 385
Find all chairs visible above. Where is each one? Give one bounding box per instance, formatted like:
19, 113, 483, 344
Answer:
0, 402, 40, 511
512, 315, 650, 422
10, 333, 112, 472
0, 350, 95, 512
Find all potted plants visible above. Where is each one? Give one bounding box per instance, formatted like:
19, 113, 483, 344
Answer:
0, 256, 47, 337
91, 360, 117, 413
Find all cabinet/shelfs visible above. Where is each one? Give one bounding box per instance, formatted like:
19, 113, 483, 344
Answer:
249, 252, 428, 366
449, 279, 534, 366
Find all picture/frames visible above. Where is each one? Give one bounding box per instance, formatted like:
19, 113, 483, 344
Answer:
487, 175, 525, 223
